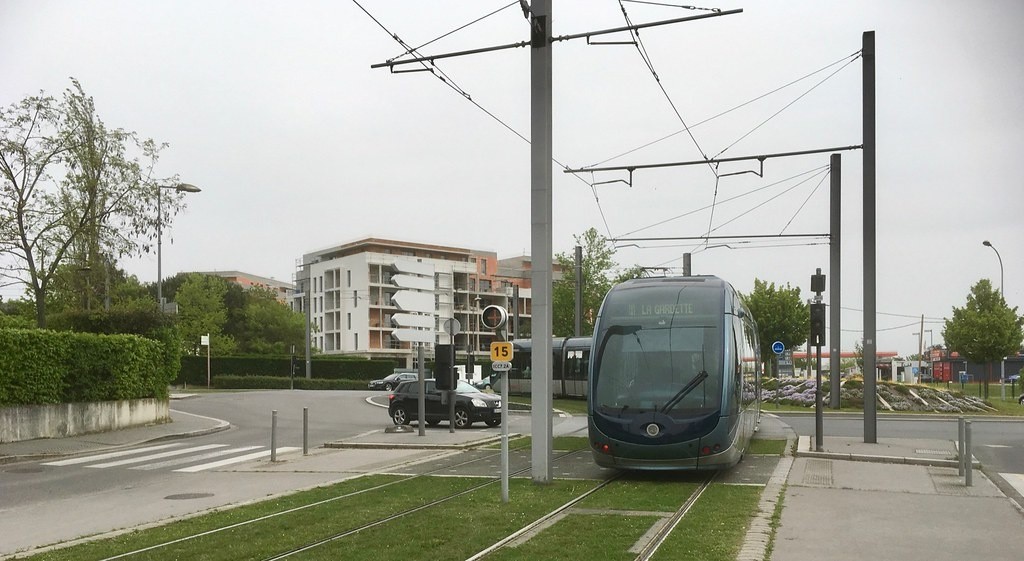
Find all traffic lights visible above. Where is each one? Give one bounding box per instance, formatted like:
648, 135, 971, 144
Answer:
480, 304, 508, 331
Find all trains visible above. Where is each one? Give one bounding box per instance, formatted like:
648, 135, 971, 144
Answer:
491, 251, 763, 473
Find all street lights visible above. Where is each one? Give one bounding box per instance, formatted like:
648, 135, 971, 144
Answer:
912, 332, 920, 353
924, 329, 934, 384
79, 266, 93, 310
156, 182, 201, 311
983, 239, 1004, 308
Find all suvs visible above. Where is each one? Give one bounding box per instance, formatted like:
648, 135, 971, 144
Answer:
387, 377, 501, 429
368, 370, 418, 392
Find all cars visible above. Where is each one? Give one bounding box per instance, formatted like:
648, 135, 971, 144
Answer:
918, 374, 934, 384
1004, 373, 1020, 383
